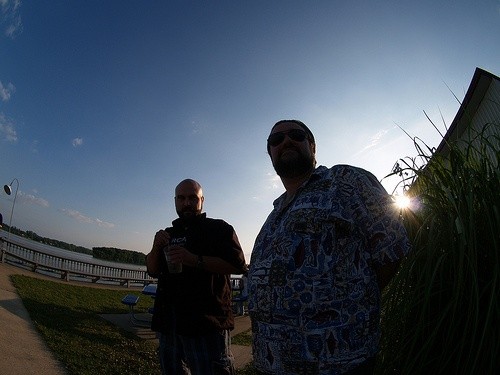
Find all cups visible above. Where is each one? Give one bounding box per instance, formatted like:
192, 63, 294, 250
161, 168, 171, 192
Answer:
164, 244, 183, 273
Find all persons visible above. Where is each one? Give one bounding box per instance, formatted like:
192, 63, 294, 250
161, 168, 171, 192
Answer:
238, 266, 250, 316
246, 117, 417, 374
143, 179, 247, 374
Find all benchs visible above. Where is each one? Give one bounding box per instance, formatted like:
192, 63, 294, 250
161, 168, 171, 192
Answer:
121, 294, 150, 330
232, 297, 242, 315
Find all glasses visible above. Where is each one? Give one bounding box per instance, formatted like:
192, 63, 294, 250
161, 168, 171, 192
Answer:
268, 128, 308, 146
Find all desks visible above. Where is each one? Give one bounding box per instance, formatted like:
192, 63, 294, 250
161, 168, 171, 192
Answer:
143, 283, 158, 300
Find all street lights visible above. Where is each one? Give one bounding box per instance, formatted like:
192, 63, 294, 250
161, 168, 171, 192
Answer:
4, 178, 21, 263
